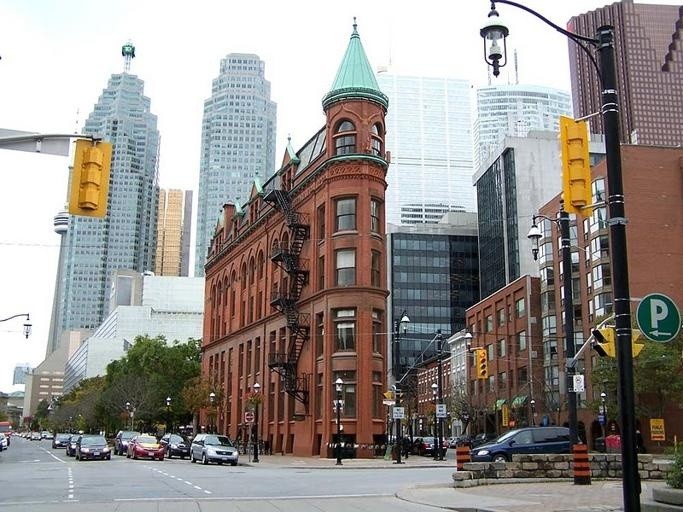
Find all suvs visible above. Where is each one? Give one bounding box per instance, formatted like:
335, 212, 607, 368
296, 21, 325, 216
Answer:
157, 433, 192, 459
112, 430, 140, 457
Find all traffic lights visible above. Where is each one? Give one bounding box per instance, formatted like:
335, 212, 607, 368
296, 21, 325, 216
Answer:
632, 329, 644, 358
476, 350, 488, 378
591, 328, 614, 358
381, 390, 393, 402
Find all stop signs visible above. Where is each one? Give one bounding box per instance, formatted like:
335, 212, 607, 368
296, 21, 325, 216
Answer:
245, 412, 254, 422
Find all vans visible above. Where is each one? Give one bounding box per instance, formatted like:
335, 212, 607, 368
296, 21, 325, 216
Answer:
188, 433, 239, 466
469, 424, 584, 464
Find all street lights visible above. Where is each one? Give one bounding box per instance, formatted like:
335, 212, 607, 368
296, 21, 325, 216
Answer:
393, 308, 410, 464
252, 382, 262, 464
0, 312, 32, 339
332, 376, 345, 466
527, 196, 579, 456
125, 401, 136, 431
208, 392, 216, 433
477, 1, 641, 512
164, 396, 172, 432
528, 397, 537, 426
436, 326, 472, 460
430, 381, 439, 461
599, 391, 608, 452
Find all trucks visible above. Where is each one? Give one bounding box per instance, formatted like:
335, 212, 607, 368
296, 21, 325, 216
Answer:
0, 421, 11, 445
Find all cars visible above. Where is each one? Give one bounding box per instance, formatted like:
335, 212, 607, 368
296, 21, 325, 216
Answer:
65, 435, 79, 456
10, 430, 54, 441
125, 432, 165, 461
51, 432, 70, 449
0, 432, 7, 451
74, 435, 111, 462
390, 431, 494, 457
